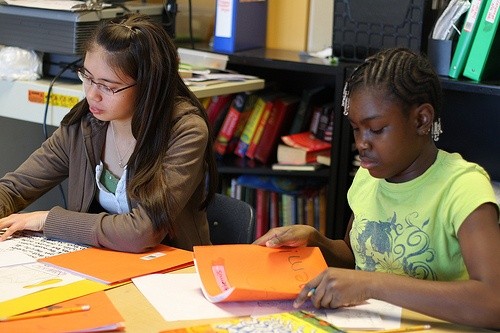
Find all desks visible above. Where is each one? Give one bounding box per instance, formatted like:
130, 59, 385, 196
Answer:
0, 78, 266, 215
0, 231, 500, 333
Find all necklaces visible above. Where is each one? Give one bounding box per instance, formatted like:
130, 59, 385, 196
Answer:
107, 120, 136, 167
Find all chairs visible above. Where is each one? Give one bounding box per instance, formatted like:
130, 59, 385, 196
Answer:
207, 193, 255, 245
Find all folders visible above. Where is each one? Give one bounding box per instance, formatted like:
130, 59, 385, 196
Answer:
212, 0, 268, 54
449, 0, 500, 87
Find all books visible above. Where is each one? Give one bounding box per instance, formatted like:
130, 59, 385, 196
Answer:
34, 236, 197, 289
0, 287, 132, 333
189, 92, 335, 171
188, 236, 335, 305
208, 163, 336, 256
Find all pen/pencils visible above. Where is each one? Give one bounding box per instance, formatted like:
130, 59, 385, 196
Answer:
0, 305, 91, 322
308, 288, 315, 297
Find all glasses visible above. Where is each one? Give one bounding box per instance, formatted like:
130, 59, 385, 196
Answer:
77, 67, 136, 95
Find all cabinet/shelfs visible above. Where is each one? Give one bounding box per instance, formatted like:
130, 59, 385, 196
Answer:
176, 52, 500, 241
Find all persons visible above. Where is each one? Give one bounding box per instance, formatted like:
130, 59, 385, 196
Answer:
1, 13, 215, 252
250, 46, 500, 329
